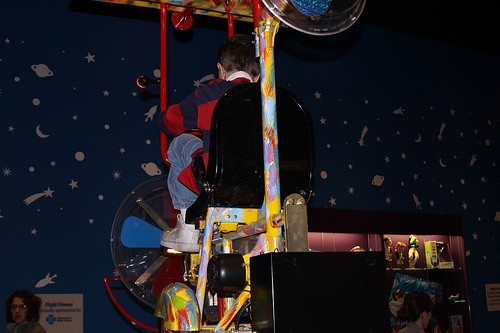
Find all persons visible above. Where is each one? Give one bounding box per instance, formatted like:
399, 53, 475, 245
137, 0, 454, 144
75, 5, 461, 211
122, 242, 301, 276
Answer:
395, 242, 406, 269
383, 238, 394, 269
157, 35, 262, 252
395, 291, 431, 333
408, 235, 421, 270
5, 290, 47, 333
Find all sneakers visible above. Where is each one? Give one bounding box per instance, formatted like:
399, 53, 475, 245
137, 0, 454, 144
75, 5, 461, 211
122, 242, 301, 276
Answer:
160, 215, 201, 252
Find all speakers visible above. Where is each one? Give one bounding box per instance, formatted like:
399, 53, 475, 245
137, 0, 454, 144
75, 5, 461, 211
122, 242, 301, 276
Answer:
248, 250, 389, 333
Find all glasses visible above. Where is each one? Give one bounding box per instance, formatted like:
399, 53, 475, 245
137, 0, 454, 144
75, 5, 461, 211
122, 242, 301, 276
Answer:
11, 304, 28, 311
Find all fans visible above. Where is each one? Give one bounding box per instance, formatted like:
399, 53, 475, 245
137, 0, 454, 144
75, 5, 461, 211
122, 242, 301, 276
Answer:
262, 0, 366, 35
110, 175, 168, 309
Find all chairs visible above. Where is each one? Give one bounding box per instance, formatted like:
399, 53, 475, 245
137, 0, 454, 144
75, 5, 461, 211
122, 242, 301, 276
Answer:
185, 82, 314, 229
